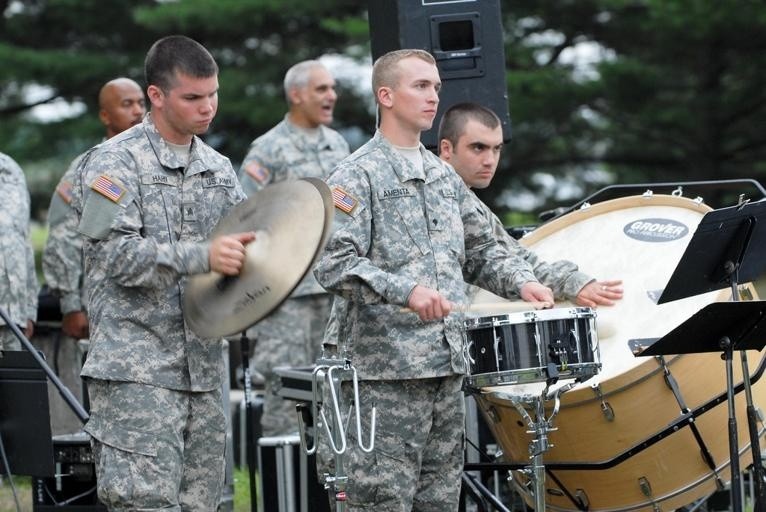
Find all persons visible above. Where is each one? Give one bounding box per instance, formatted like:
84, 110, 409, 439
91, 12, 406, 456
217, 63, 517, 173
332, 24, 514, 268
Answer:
311, 48, 556, 512
0, 150, 40, 353
437, 102, 624, 311
75, 35, 258, 512
42, 76, 148, 342
239, 59, 352, 446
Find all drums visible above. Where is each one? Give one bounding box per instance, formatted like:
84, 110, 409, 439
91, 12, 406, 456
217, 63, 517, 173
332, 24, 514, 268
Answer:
467, 185, 766, 512
461, 306, 602, 392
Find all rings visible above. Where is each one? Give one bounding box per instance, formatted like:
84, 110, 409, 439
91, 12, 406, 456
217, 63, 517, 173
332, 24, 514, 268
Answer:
602, 284, 608, 291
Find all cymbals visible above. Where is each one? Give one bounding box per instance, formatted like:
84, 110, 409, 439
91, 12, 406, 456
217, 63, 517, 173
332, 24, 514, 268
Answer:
185, 178, 334, 339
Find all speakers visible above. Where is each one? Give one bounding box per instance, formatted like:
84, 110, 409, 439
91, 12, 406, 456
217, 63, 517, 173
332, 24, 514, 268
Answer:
367, 0, 513, 149
